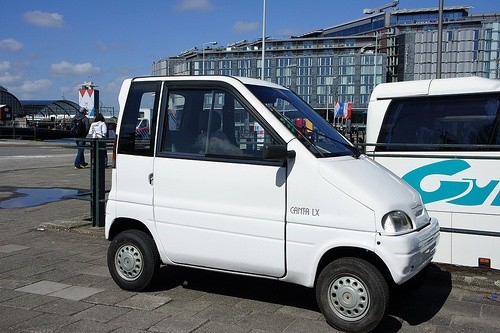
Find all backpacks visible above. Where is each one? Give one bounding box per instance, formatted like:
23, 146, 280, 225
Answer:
73, 117, 86, 138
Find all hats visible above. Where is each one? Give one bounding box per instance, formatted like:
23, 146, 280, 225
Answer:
79, 108, 87, 112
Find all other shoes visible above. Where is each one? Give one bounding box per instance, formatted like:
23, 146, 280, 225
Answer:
75, 166, 82, 169
82, 163, 88, 166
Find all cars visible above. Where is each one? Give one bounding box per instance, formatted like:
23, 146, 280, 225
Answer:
105, 76, 500, 333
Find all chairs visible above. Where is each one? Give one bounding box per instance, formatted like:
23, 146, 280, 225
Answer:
198, 110, 244, 156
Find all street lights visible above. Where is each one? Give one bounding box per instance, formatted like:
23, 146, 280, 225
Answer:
203, 42, 217, 75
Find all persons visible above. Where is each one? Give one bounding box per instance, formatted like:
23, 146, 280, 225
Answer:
70, 107, 89, 170
88, 113, 107, 167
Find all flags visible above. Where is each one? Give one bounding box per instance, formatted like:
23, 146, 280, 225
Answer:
333, 102, 351, 119
